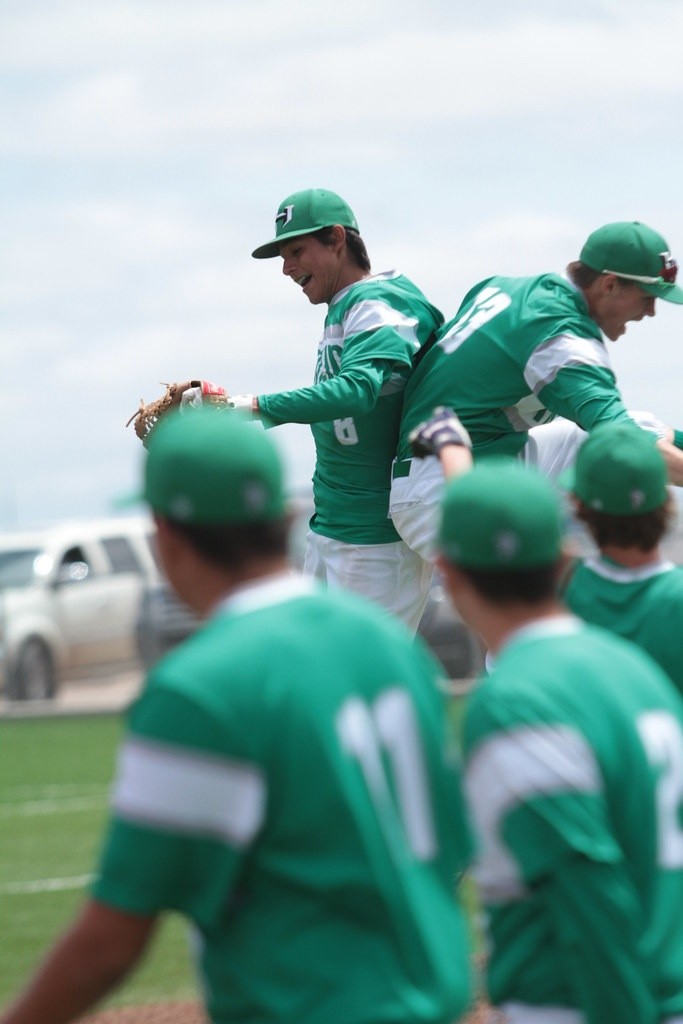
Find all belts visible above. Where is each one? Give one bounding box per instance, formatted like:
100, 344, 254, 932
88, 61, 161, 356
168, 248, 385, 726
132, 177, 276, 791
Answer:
392, 457, 446, 479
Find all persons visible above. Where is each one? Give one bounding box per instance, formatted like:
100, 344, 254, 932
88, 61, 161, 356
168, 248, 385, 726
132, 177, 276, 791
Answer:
0, 396, 485, 1024
406, 403, 683, 706
385, 220, 682, 679
133, 186, 450, 655
430, 454, 682, 1024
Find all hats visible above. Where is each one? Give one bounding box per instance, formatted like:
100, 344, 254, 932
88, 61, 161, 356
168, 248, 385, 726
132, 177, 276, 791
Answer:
145, 406, 285, 522
252, 187, 360, 260
561, 423, 668, 515
579, 221, 683, 305
440, 461, 561, 567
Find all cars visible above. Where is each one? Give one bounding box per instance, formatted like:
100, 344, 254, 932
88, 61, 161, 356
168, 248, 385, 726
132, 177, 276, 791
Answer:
1, 512, 159, 703
